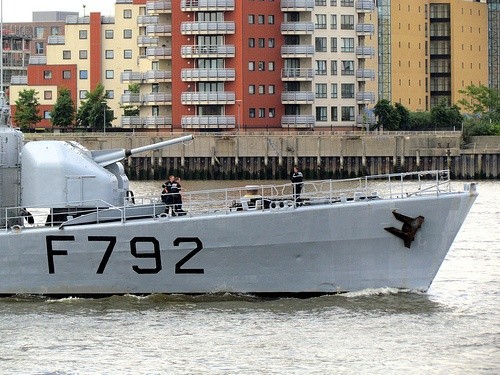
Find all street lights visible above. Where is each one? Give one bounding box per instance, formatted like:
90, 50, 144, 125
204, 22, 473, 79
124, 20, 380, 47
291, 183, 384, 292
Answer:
236, 99, 243, 132
101, 102, 107, 132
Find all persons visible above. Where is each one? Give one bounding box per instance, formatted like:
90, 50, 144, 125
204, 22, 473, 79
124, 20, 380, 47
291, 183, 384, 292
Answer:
291, 166, 303, 207
162, 175, 176, 216
170, 177, 183, 216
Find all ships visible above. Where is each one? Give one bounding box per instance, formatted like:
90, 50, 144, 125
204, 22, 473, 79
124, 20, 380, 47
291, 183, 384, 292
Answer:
0, 117, 479, 294
